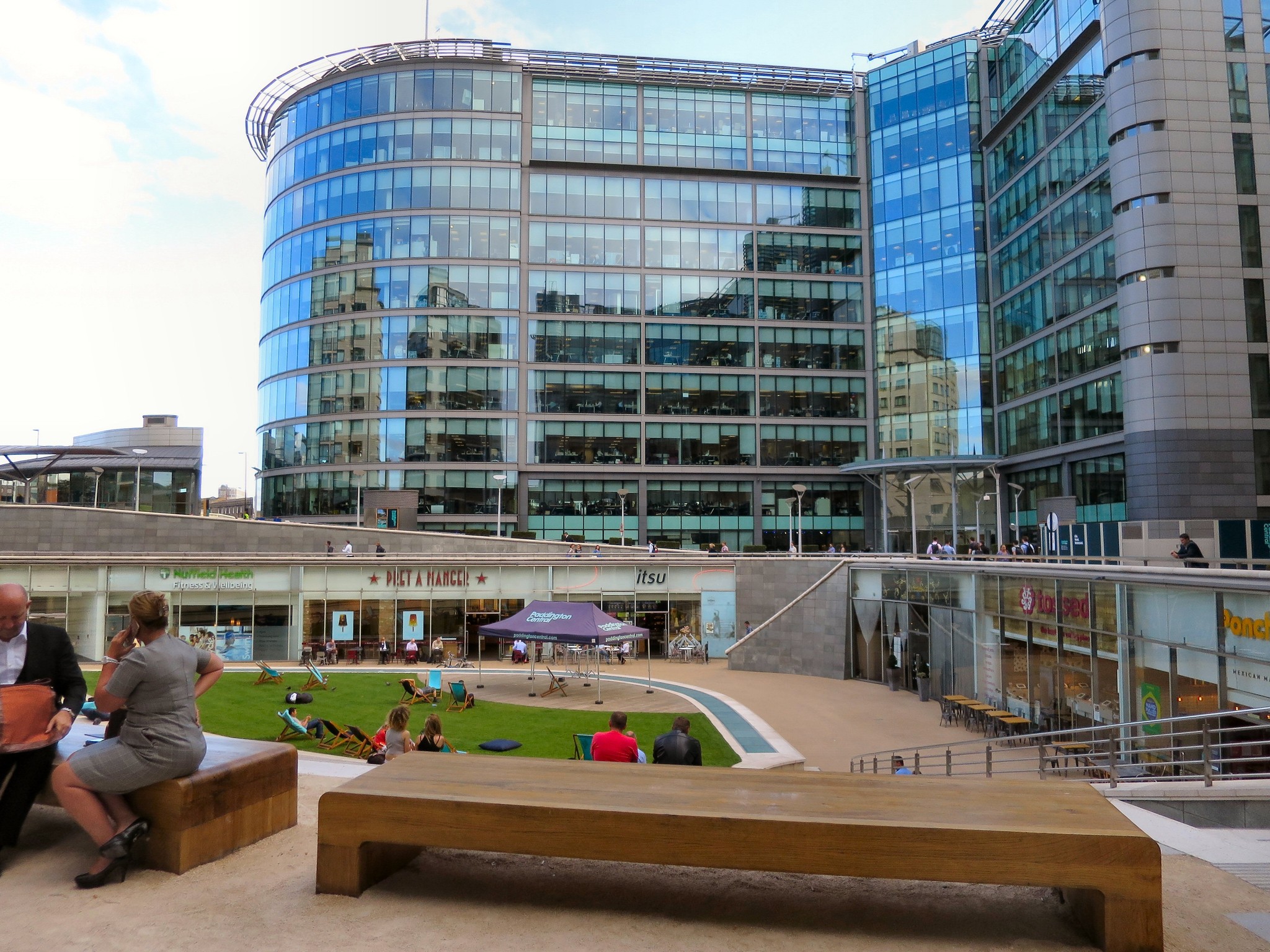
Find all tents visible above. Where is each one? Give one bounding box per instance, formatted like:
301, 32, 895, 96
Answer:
477, 599, 653, 705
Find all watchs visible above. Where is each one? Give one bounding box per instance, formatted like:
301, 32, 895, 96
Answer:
60, 707, 75, 718
101, 656, 119, 665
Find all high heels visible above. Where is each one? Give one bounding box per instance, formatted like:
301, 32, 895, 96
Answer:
99, 816, 150, 858
74, 851, 132, 887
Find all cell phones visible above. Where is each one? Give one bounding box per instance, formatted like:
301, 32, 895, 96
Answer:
123, 619, 139, 649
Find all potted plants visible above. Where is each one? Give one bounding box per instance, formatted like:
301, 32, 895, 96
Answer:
916, 672, 930, 702
885, 654, 901, 692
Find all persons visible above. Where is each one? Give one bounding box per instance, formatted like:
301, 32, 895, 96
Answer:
243, 508, 249, 519
788, 542, 797, 557
288, 708, 326, 740
180, 629, 235, 653
511, 639, 529, 664
595, 640, 630, 665
707, 542, 729, 557
399, 678, 440, 703
590, 711, 702, 766
1171, 533, 1209, 568
894, 756, 912, 775
0, 584, 88, 861
648, 539, 656, 557
927, 535, 1035, 564
745, 621, 753, 635
326, 539, 386, 558
828, 544, 845, 557
51, 589, 224, 886
563, 532, 602, 558
459, 680, 475, 706
320, 637, 443, 665
374, 705, 445, 762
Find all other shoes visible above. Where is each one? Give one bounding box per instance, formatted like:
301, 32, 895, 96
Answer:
315, 734, 326, 740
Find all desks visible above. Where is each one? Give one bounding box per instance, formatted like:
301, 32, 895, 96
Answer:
968, 704, 997, 733
943, 694, 969, 714
985, 710, 1015, 738
1000, 717, 1031, 748
568, 648, 583, 664
682, 645, 696, 661
433, 256, 820, 516
679, 648, 693, 664
605, 648, 620, 665
1090, 759, 1152, 778
954, 699, 985, 728
1051, 741, 1091, 778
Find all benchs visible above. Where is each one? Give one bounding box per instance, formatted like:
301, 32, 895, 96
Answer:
313, 752, 1163, 952
34, 715, 299, 878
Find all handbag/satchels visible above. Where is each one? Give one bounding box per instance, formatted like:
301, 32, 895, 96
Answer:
0, 679, 63, 754
368, 754, 385, 764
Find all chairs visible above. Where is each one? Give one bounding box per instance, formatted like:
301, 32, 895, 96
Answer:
569, 733, 595, 760
512, 644, 632, 697
254, 648, 476, 758
940, 692, 1165, 782
669, 643, 704, 664
